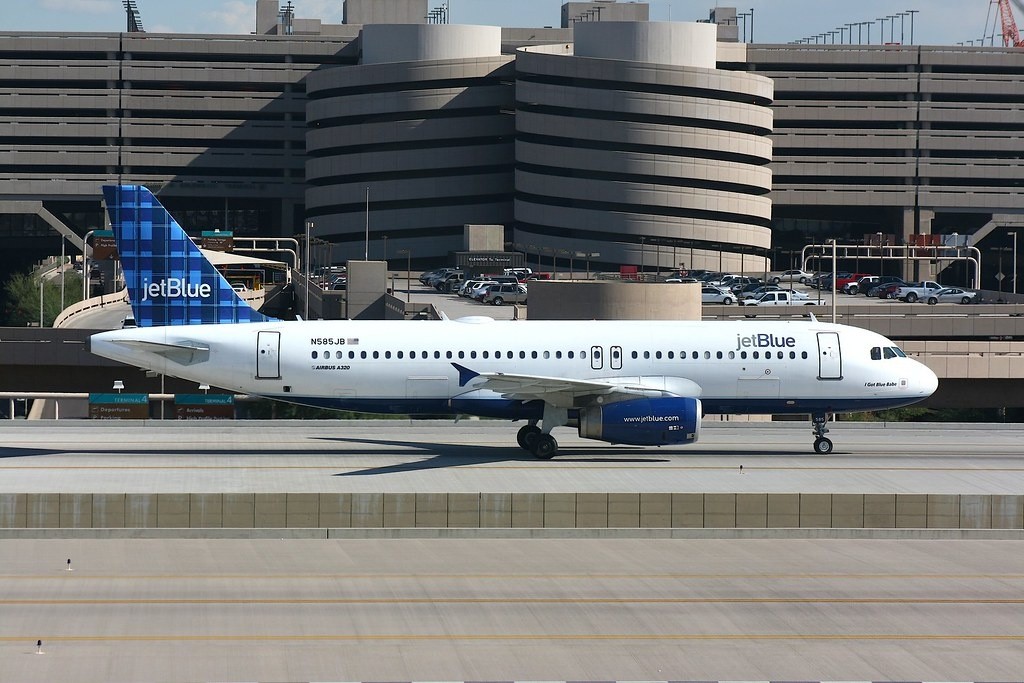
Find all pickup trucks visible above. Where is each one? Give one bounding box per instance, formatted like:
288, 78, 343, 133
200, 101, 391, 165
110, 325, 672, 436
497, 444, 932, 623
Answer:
892, 280, 944, 303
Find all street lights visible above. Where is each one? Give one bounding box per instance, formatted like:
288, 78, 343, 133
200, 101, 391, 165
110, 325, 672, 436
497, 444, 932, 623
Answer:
60, 234, 73, 314
363, 185, 370, 262
399, 248, 411, 303
875, 231, 884, 280
1007, 231, 1018, 293
381, 234, 389, 263
825, 237, 837, 325
639, 236, 647, 275
649, 238, 802, 290
303, 221, 314, 320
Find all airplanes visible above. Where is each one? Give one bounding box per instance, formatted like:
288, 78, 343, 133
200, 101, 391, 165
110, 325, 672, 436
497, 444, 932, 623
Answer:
86, 179, 941, 461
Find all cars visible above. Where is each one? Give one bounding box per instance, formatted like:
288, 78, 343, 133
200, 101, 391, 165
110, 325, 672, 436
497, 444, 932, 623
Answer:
918, 287, 976, 306
769, 270, 814, 284
664, 269, 907, 318
417, 265, 551, 306
302, 265, 347, 291
